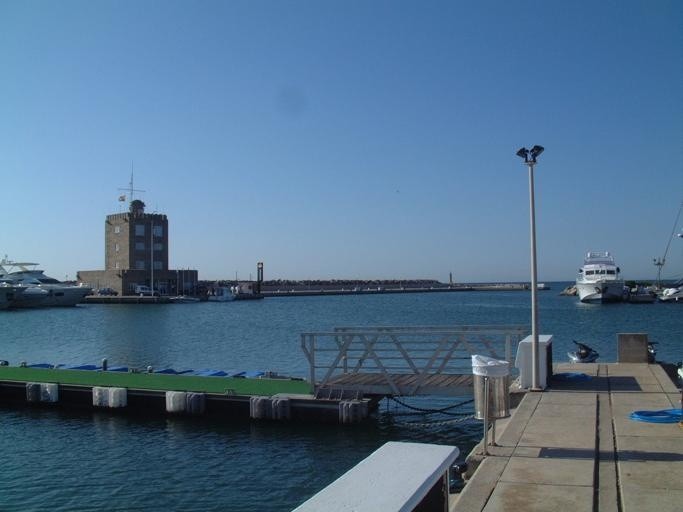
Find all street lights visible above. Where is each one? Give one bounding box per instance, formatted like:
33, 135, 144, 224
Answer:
517, 144, 551, 391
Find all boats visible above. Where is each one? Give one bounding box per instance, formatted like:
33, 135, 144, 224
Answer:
0, 257, 92, 309
575, 250, 623, 303
624, 281, 681, 303
208, 287, 236, 301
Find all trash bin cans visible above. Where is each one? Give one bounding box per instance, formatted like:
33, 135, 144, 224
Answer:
471, 353, 510, 456
518, 334, 553, 388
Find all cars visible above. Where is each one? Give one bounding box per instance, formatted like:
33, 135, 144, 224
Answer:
97, 288, 118, 297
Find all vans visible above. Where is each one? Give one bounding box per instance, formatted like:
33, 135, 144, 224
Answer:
135, 285, 160, 295
